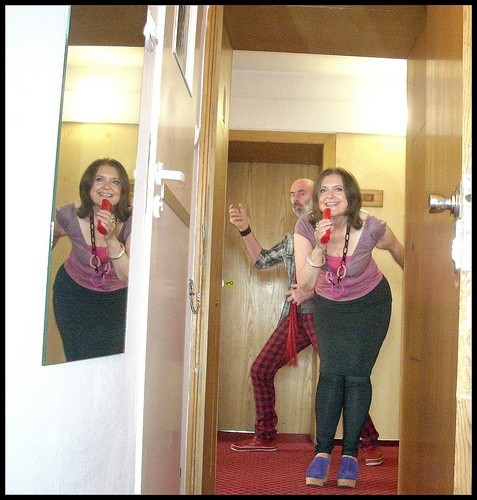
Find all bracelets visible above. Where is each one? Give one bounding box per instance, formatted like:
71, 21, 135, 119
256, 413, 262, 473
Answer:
305, 250, 326, 268
239, 226, 251, 235
106, 244, 126, 259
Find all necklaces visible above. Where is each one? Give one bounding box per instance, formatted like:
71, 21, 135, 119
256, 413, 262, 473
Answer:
87, 209, 112, 289
322, 220, 351, 297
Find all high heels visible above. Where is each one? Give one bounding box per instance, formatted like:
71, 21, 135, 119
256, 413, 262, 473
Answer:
305, 456, 331, 486
337, 457, 359, 487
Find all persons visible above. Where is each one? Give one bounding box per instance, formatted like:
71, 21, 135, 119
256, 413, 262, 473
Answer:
294, 166, 406, 488
230, 178, 384, 465
50, 157, 133, 361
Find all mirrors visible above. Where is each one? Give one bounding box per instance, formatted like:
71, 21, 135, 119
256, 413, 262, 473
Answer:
42, 4, 174, 366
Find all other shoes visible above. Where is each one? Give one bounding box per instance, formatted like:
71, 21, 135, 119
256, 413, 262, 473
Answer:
366, 448, 385, 467
230, 437, 278, 452
